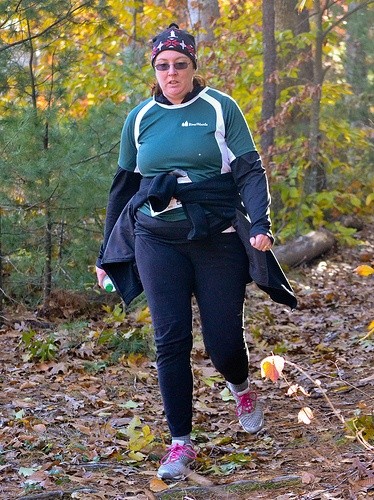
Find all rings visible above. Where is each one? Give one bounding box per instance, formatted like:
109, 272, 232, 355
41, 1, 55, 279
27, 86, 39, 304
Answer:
264, 246, 269, 249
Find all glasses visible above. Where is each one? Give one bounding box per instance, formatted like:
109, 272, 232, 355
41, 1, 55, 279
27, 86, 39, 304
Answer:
154, 60, 193, 71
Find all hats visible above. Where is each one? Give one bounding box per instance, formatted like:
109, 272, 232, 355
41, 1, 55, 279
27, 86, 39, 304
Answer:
150, 23, 198, 70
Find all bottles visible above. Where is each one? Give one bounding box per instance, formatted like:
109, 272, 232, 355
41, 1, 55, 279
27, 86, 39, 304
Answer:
102, 275, 117, 293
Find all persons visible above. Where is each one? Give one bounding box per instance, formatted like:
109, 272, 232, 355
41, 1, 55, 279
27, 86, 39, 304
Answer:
94, 24, 297, 480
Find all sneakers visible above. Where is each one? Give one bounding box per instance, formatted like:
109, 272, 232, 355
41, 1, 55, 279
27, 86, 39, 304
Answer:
226, 378, 264, 434
157, 440, 196, 480
